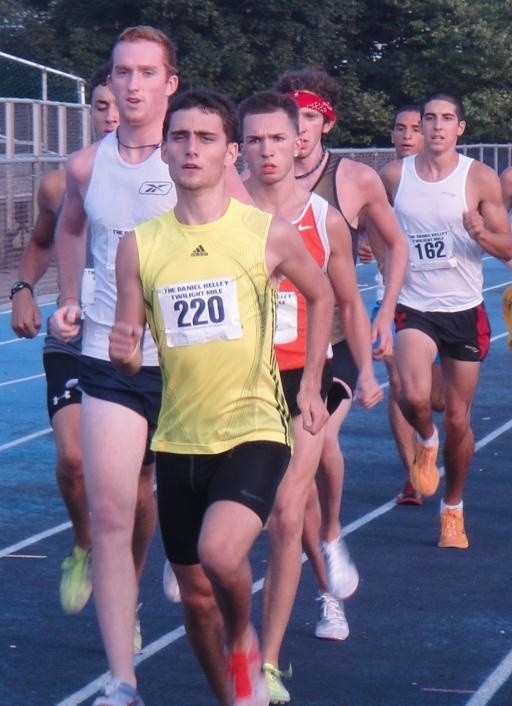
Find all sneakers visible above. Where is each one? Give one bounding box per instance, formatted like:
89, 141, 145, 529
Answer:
91, 679, 146, 706
319, 526, 359, 599
395, 480, 423, 505
59, 544, 93, 614
437, 499, 469, 549
222, 622, 292, 706
316, 591, 351, 639
410, 424, 440, 496
